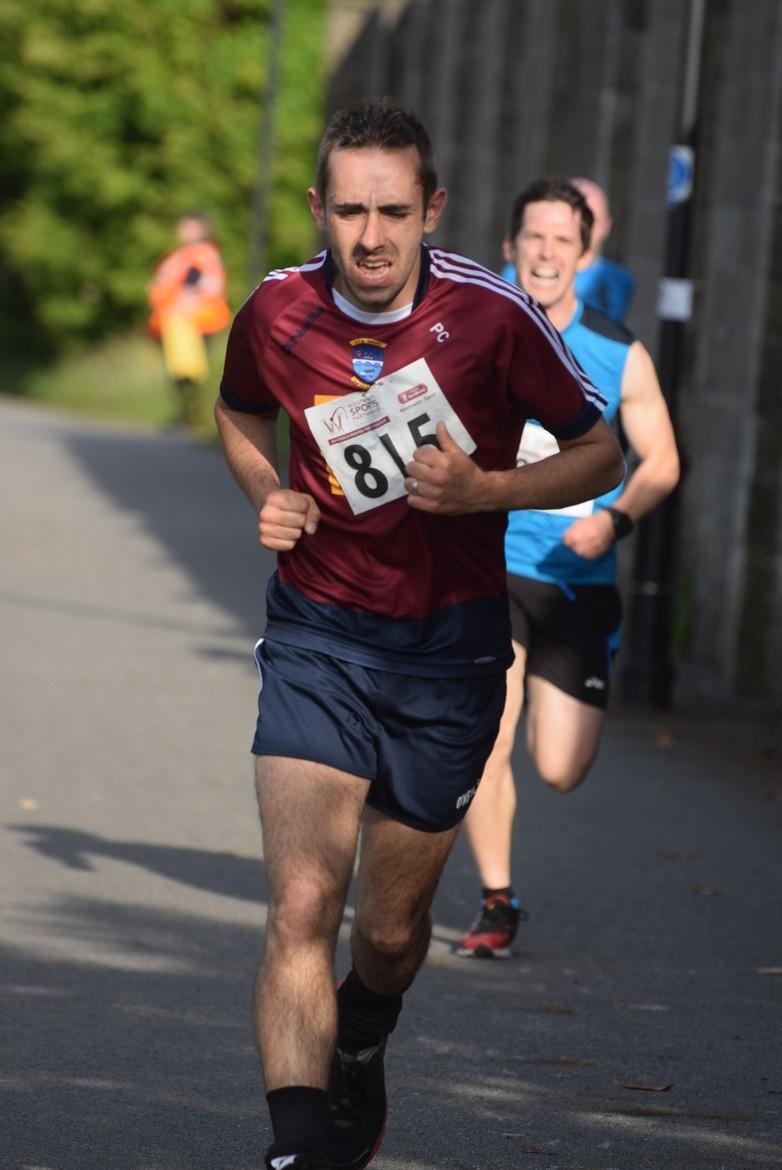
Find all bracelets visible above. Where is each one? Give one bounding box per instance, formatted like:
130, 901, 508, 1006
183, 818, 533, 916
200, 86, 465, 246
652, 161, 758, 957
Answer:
602, 504, 634, 542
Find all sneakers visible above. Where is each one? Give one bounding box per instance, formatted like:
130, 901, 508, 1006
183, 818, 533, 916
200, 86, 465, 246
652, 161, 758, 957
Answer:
457, 895, 530, 958
261, 1132, 339, 1170
323, 1036, 390, 1170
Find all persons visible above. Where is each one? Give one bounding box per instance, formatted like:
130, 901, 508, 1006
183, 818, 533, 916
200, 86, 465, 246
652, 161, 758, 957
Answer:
211, 100, 626, 1170
146, 215, 232, 438
451, 176, 679, 958
496, 171, 636, 327
